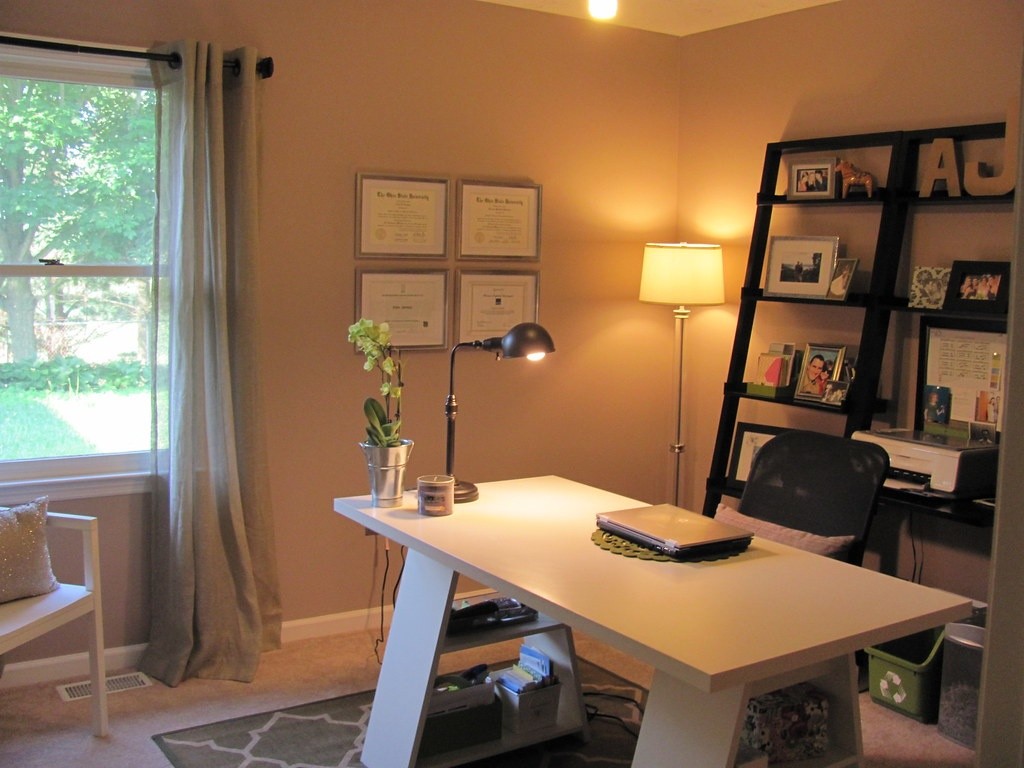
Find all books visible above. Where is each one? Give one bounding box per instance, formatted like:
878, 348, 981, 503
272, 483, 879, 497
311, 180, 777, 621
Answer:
756, 343, 800, 386
496, 645, 559, 694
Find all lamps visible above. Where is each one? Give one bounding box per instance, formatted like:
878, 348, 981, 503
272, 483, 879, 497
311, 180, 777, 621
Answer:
446, 323, 557, 503
639, 242, 726, 504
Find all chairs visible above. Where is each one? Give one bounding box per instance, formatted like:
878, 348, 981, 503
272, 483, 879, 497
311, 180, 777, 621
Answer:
736, 430, 889, 568
0, 510, 114, 737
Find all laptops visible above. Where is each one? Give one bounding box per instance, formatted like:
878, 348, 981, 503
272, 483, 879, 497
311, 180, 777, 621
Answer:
596, 503, 755, 558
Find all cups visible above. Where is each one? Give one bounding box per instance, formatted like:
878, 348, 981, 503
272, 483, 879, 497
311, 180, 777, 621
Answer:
417, 475, 454, 514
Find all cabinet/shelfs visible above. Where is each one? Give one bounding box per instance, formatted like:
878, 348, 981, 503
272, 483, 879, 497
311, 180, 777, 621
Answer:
699, 122, 1024, 696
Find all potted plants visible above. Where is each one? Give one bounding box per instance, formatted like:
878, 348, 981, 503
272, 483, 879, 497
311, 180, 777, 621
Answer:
347, 320, 415, 507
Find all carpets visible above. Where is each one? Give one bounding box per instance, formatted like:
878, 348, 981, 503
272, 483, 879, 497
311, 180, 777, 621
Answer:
152, 651, 668, 767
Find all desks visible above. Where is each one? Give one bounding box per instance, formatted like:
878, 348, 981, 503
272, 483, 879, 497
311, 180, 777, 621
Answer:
333, 472, 974, 768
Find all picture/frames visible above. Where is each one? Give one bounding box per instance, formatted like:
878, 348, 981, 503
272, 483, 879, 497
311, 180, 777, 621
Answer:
793, 341, 850, 407
825, 258, 860, 302
786, 157, 836, 199
943, 259, 1012, 313
763, 234, 840, 298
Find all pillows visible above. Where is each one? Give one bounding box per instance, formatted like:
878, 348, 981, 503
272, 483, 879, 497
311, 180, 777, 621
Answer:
0, 494, 62, 604
715, 504, 855, 559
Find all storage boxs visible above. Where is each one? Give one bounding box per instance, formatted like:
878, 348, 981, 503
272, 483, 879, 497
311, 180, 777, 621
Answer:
861, 628, 949, 725
488, 666, 562, 734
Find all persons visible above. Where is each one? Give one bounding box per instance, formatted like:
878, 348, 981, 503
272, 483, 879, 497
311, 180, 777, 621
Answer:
960, 275, 997, 300
987, 396, 1000, 422
798, 170, 827, 191
924, 392, 944, 423
795, 261, 803, 282
831, 263, 851, 296
801, 354, 846, 401
